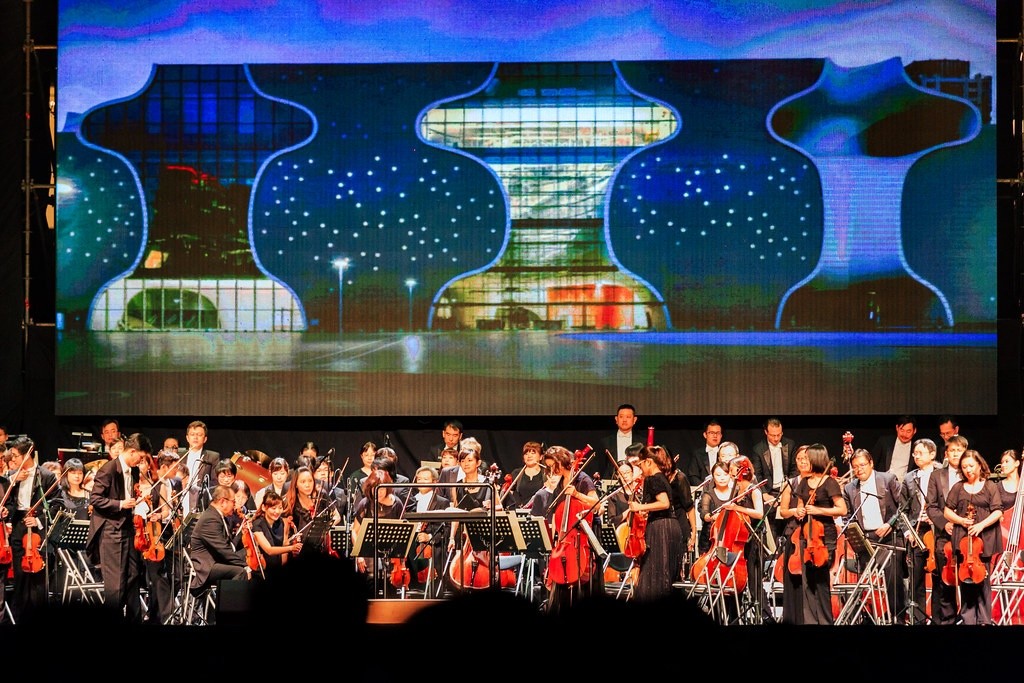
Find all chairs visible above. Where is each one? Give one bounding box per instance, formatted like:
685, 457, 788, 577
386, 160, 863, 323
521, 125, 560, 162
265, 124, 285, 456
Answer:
57, 547, 1024, 625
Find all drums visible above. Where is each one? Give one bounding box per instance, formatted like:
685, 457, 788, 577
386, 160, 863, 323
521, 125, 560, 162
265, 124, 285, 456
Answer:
231, 451, 273, 509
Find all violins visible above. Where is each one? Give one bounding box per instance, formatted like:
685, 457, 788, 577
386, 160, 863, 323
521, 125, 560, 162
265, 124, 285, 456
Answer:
922, 503, 987, 587
0, 520, 13, 565
389, 555, 411, 588
415, 521, 438, 584
623, 478, 648, 559
788, 489, 829, 576
286, 514, 301, 558
309, 506, 339, 559
235, 506, 266, 571
133, 483, 182, 563
20, 506, 46, 573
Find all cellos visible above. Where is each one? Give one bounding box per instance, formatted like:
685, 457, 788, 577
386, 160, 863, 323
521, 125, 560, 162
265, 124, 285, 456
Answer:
988, 450, 1024, 626
449, 462, 517, 594
547, 442, 596, 587
689, 459, 751, 595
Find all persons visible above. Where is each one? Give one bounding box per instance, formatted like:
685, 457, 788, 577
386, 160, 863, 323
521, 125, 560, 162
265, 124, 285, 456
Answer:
944, 450, 1004, 626
189, 486, 252, 598
796, 443, 848, 625
0, 418, 1024, 626
86, 432, 153, 622
589, 403, 648, 479
628, 445, 684, 599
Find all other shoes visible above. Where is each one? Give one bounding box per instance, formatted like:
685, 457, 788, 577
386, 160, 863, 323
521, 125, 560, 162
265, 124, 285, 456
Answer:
763, 614, 777, 624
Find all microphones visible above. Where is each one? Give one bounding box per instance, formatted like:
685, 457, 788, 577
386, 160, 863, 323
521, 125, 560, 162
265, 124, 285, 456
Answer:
196, 459, 212, 466
861, 491, 883, 499
354, 478, 365, 498
386, 434, 391, 447
348, 479, 355, 503
914, 479, 929, 504
618, 478, 630, 501
198, 476, 207, 500
785, 476, 795, 493
32, 466, 39, 488
338, 470, 348, 495
315, 449, 334, 469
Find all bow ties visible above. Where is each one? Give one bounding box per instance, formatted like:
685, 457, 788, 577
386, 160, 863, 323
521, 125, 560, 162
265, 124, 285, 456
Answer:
706, 446, 718, 453
444, 445, 458, 451
917, 465, 933, 478
619, 431, 630, 438
124, 467, 131, 473
29, 469, 35, 476
859, 477, 871, 486
769, 441, 782, 450
416, 494, 430, 501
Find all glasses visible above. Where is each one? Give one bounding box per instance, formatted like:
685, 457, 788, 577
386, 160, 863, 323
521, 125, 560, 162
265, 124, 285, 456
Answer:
219, 497, 236, 506
911, 452, 929, 457
851, 463, 868, 471
768, 432, 783, 438
10, 453, 22, 459
795, 457, 808, 463
620, 470, 632, 475
364, 453, 375, 458
708, 431, 721, 436
940, 429, 955, 437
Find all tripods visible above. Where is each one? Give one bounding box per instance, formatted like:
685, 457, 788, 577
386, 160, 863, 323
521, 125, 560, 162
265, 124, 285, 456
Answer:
162, 580, 209, 626
731, 482, 788, 624
897, 548, 937, 627
